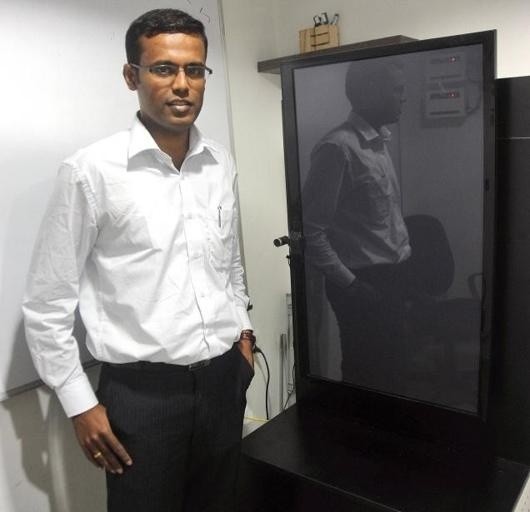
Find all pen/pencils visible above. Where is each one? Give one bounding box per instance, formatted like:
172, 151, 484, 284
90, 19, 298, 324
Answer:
217, 206, 223, 228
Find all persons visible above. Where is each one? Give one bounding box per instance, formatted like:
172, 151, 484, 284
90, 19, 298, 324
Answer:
20, 9, 254, 512
303, 56, 412, 392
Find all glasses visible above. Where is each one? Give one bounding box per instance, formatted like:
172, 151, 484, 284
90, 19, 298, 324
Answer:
127, 60, 213, 84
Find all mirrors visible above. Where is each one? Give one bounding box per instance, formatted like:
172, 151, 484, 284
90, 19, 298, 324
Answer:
280, 29, 496, 427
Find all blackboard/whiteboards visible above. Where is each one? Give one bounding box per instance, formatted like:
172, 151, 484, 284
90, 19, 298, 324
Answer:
0, 0, 250, 402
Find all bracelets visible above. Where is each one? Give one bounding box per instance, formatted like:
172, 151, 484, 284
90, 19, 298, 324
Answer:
241, 333, 256, 348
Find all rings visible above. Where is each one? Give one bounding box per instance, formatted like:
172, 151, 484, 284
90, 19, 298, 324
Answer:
93, 451, 102, 459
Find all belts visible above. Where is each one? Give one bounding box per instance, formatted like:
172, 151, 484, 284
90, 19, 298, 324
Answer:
102, 342, 239, 374
